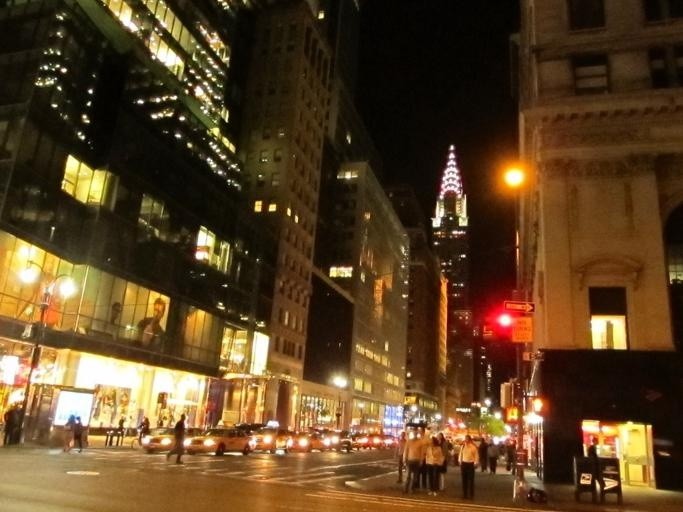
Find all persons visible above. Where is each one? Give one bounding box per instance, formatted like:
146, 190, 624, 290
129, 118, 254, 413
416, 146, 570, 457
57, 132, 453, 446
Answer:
166, 412, 186, 466
573, 435, 605, 504
63, 413, 73, 452
90, 302, 122, 336
393, 425, 514, 503
155, 416, 163, 427
118, 416, 125, 433
138, 417, 148, 442
72, 416, 83, 452
1, 406, 20, 445
130, 297, 164, 351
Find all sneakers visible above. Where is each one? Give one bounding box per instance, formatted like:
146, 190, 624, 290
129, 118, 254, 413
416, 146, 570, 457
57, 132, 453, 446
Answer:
428, 491, 437, 496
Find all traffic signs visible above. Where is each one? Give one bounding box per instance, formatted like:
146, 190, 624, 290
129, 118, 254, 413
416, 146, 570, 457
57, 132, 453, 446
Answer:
504, 300, 536, 314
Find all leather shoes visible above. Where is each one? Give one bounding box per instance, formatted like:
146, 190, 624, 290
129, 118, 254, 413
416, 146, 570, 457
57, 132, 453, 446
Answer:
464, 495, 473, 499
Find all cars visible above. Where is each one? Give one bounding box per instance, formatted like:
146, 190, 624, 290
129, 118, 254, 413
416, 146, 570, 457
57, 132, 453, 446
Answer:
184, 428, 256, 456
142, 426, 203, 453
235, 423, 396, 454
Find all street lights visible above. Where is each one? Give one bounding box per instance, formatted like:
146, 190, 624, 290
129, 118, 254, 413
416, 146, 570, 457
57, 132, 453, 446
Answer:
229, 351, 252, 424
332, 375, 347, 430
532, 399, 543, 476
496, 314, 523, 450
17, 245, 78, 440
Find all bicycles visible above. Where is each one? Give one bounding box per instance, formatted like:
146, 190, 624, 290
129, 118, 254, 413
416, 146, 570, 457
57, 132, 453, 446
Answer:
131, 427, 146, 450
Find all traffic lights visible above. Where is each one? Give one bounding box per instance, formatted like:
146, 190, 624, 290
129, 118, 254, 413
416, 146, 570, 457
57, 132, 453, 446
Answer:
525, 397, 548, 415
